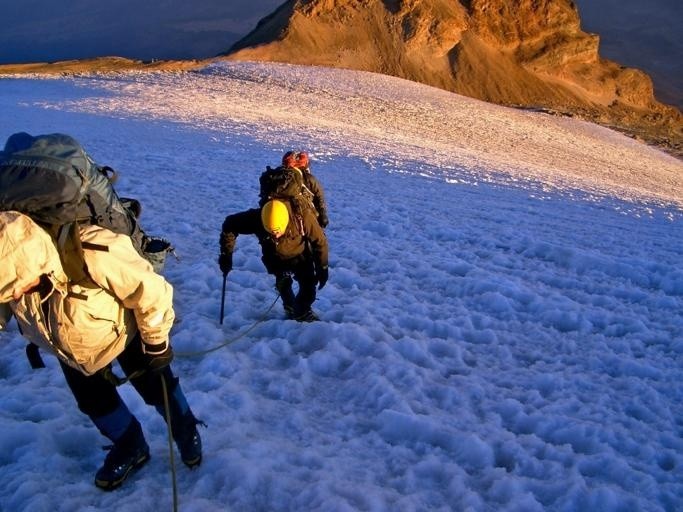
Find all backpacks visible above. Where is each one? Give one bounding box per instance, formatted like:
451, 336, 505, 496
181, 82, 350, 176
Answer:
0, 130, 171, 278
257, 162, 320, 221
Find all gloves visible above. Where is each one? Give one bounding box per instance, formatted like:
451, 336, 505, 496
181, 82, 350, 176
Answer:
315, 264, 328, 290
216, 251, 233, 276
140, 339, 174, 373
317, 207, 329, 228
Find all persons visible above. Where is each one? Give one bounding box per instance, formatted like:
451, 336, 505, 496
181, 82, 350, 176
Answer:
281, 150, 327, 229
0, 208, 207, 490
219, 205, 328, 324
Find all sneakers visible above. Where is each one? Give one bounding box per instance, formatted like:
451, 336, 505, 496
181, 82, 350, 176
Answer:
172, 425, 200, 466
94, 439, 153, 486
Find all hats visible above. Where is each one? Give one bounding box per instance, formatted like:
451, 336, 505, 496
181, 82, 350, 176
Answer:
261, 200, 290, 237
